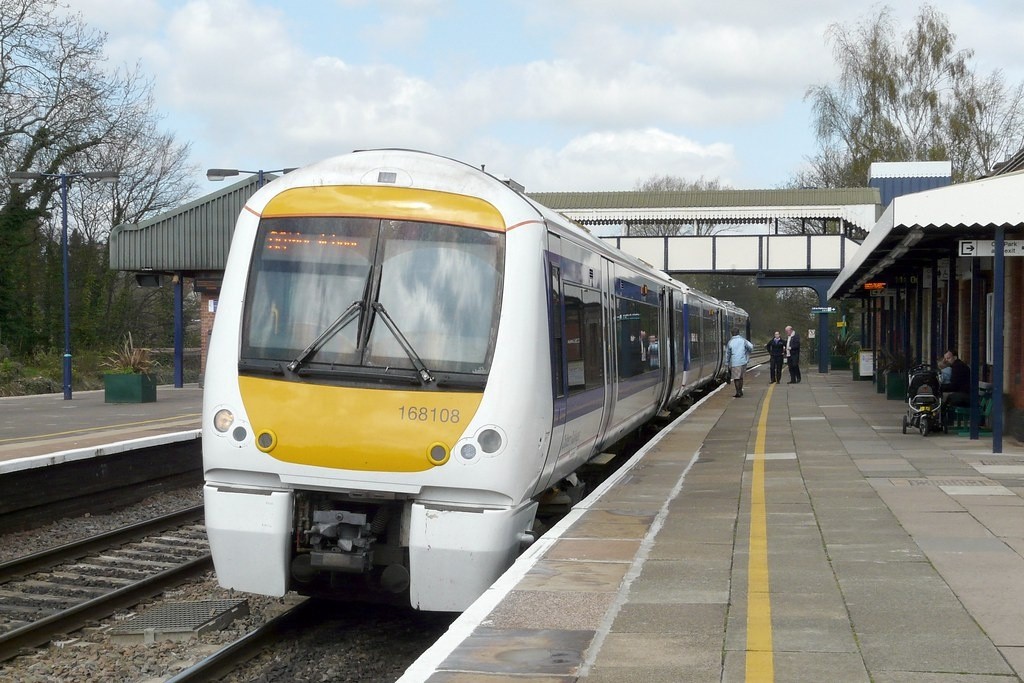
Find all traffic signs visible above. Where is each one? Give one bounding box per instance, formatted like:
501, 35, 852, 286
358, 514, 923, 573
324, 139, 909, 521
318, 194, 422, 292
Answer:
958, 239, 1024, 256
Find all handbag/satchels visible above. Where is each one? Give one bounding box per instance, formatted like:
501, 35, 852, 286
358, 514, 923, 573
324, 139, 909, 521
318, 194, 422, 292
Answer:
721, 367, 731, 384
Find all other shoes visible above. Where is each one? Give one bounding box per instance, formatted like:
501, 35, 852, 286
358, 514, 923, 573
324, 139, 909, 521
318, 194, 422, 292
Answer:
770, 381, 776, 384
776, 379, 780, 384
796, 377, 801, 383
787, 382, 797, 384
733, 392, 744, 397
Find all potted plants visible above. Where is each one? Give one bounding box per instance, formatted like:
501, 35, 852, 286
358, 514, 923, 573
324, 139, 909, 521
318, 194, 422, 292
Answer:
876, 342, 917, 400
828, 332, 856, 371
103, 331, 157, 403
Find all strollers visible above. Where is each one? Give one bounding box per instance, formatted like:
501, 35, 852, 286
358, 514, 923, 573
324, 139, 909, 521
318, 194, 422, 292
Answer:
903, 364, 942, 437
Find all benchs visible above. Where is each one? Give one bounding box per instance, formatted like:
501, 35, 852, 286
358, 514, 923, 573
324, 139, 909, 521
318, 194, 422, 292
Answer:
944, 380, 994, 437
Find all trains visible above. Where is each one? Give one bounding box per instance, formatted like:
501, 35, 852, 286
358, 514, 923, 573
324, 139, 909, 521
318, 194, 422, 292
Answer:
203, 149, 752, 613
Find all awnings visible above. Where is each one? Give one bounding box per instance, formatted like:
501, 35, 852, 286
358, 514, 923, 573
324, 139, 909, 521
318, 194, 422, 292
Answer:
827, 171, 1024, 302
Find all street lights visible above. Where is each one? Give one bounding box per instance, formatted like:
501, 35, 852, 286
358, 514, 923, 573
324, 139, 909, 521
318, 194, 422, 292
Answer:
7, 171, 120, 400
206, 166, 298, 190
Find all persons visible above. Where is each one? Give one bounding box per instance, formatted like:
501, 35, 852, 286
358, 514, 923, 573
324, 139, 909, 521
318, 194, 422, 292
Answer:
785, 325, 801, 384
766, 331, 786, 385
725, 328, 754, 398
641, 330, 658, 372
939, 349, 970, 431
